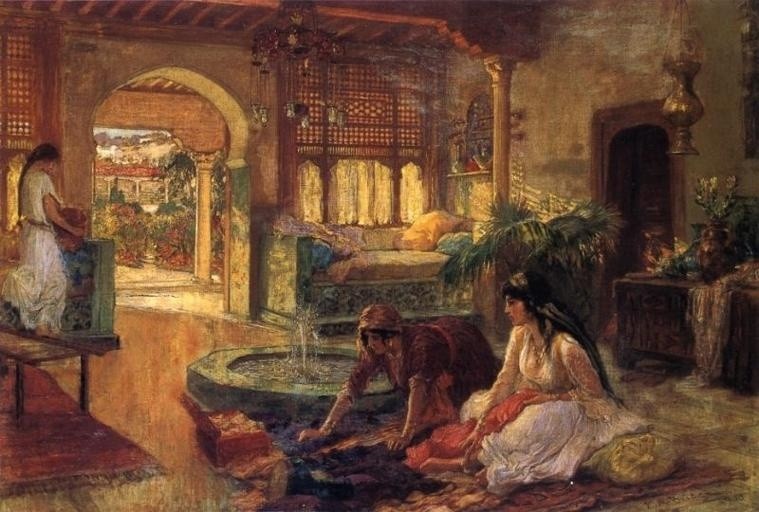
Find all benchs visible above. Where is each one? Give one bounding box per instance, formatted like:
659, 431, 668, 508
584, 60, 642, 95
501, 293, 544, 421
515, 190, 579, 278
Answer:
0, 323, 120, 419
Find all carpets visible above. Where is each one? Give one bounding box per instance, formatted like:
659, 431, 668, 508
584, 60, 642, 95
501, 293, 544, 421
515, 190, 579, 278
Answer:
176, 390, 753, 510
0, 354, 173, 498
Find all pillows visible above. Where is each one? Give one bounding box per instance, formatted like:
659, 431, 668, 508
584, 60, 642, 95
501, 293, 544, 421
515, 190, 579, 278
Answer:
399, 210, 463, 252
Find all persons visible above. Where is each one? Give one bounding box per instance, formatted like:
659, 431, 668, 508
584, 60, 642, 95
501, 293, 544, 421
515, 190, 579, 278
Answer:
11, 144, 83, 338
297, 302, 497, 453
419, 268, 614, 509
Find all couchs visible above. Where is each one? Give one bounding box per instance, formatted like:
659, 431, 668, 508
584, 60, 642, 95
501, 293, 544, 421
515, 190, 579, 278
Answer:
257, 212, 491, 322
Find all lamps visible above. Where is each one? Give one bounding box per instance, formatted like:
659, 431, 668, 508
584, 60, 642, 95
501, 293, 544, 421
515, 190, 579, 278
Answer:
248, 2, 350, 132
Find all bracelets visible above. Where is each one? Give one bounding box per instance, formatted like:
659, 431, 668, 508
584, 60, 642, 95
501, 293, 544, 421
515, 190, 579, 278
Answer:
546, 393, 560, 400
